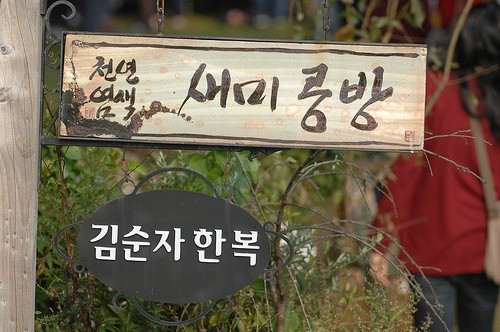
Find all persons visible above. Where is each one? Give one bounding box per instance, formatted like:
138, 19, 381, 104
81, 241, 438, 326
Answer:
370, 0, 500, 332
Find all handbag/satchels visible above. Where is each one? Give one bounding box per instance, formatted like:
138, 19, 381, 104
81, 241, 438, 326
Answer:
483, 200, 499, 286
